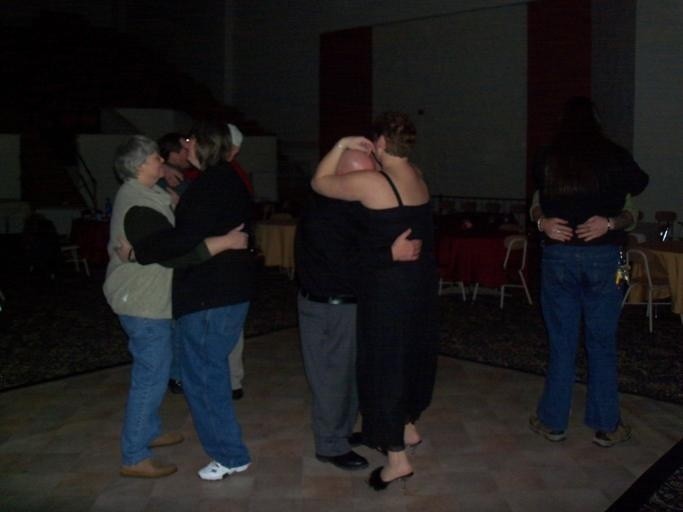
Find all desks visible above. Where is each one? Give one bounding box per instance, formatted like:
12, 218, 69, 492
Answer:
431, 224, 530, 300
628, 239, 683, 326
435, 210, 517, 234
254, 219, 297, 277
65, 212, 110, 269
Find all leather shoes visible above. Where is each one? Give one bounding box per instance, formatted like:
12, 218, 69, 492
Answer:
315, 432, 369, 471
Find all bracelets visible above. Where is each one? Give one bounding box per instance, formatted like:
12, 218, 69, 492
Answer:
607, 216, 616, 230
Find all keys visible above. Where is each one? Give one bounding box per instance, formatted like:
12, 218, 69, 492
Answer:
615, 247, 632, 290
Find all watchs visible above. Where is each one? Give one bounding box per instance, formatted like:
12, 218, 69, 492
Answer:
537, 215, 543, 232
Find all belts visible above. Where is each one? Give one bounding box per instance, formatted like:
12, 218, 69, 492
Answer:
302, 292, 362, 304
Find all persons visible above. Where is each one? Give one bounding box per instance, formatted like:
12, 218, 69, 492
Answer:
295, 149, 422, 472
102, 115, 257, 480
310, 117, 436, 491
533, 93, 650, 446
529, 101, 639, 320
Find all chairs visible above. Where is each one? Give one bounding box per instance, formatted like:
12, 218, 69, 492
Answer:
31, 215, 91, 279
271, 213, 290, 221
470, 236, 534, 308
431, 263, 468, 304
619, 248, 682, 329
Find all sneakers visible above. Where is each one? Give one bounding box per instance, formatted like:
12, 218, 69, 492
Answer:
593, 423, 631, 448
197, 460, 252, 481
148, 433, 185, 447
119, 460, 177, 478
529, 414, 565, 441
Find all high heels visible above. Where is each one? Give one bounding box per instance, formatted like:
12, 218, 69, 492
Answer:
369, 466, 414, 492
376, 436, 422, 457
